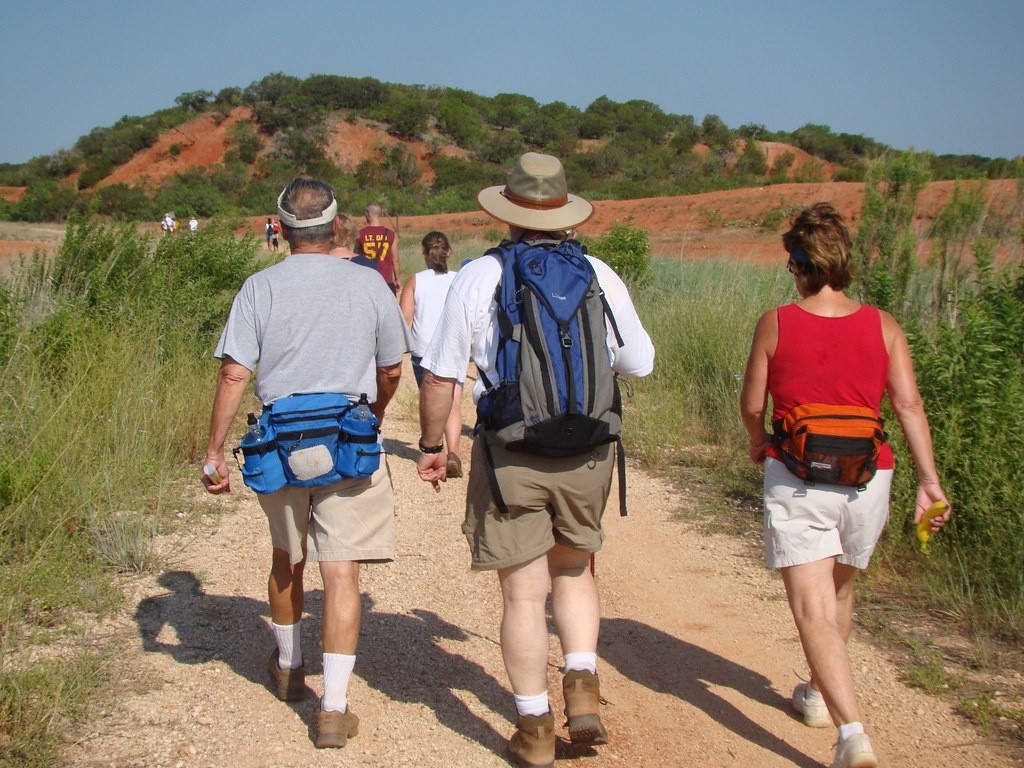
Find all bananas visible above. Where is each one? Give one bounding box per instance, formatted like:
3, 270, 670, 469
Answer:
916, 501, 950, 555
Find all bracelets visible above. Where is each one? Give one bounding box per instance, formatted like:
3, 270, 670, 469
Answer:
418, 434, 444, 454
748, 434, 772, 452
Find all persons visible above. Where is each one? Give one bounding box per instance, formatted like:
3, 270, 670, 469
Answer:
271, 218, 280, 240
198, 177, 414, 748
741, 200, 951, 768
271, 230, 279, 252
160, 210, 177, 238
265, 218, 273, 250
353, 201, 399, 298
326, 212, 380, 273
188, 216, 198, 237
396, 228, 468, 478
418, 150, 655, 768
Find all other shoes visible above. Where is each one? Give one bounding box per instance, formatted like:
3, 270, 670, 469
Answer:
446, 452, 462, 478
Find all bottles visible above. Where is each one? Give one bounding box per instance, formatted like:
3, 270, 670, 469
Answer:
348, 392, 374, 421
243, 413, 267, 444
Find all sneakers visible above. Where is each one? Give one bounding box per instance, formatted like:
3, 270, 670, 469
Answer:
834, 736, 877, 768
792, 678, 830, 728
563, 669, 609, 744
507, 703, 555, 768
314, 696, 358, 748
270, 646, 305, 702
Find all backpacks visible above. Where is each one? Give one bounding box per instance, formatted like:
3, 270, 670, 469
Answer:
483, 239, 626, 460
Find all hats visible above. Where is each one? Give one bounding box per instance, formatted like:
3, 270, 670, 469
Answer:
476, 150, 595, 232
276, 180, 337, 229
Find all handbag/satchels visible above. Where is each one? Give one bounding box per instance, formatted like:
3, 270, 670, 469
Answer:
232, 388, 382, 493
767, 404, 890, 492
